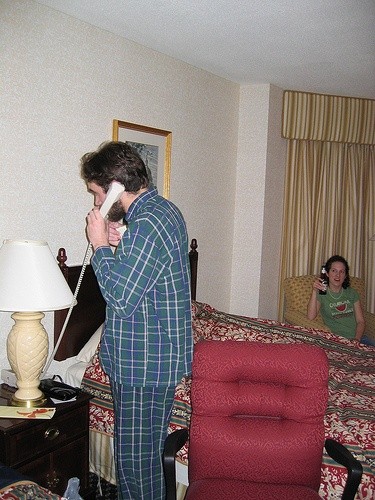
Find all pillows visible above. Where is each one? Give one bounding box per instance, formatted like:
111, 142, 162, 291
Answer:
75, 321, 103, 363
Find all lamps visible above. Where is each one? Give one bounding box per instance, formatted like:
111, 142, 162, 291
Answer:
0, 238, 78, 408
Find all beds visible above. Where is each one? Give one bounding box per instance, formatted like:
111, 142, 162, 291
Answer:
54, 237, 375, 500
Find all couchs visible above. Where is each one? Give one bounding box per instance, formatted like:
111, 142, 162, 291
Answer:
281, 274, 375, 340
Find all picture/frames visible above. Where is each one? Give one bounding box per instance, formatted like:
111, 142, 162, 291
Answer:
113, 119, 172, 202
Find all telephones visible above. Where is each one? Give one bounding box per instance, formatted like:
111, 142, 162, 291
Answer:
84, 183, 125, 218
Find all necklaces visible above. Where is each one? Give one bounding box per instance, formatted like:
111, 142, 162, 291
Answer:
328, 286, 343, 299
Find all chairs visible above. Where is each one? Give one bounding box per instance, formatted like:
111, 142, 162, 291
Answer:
163, 339, 364, 500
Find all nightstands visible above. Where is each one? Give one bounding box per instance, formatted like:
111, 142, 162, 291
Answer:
0, 379, 97, 500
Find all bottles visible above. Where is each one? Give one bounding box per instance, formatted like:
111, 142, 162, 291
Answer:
319, 264, 328, 295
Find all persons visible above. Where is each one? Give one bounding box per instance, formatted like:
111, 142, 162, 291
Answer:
80, 141, 194, 500
308, 255, 375, 348
